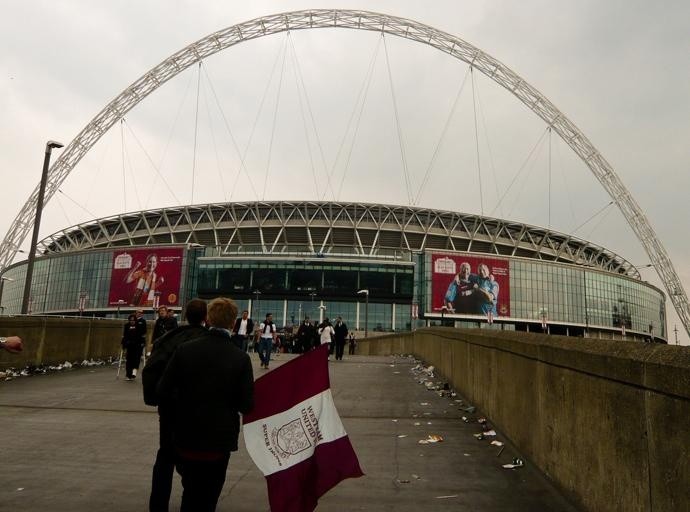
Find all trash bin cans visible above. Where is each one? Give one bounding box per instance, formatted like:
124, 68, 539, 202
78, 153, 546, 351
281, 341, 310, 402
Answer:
0, 337, 6, 347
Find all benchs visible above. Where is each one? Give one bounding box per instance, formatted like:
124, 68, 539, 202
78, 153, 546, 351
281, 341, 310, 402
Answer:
243, 342, 365, 511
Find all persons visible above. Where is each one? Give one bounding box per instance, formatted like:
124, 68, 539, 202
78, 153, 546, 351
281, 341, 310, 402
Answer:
0, 335, 23, 353
335, 317, 348, 360
153, 305, 178, 342
454, 263, 499, 316
142, 300, 208, 512
257, 313, 277, 368
153, 300, 254, 512
234, 310, 252, 349
297, 315, 313, 350
445, 263, 479, 314
126, 254, 164, 307
318, 320, 335, 361
135, 309, 146, 347
123, 315, 146, 380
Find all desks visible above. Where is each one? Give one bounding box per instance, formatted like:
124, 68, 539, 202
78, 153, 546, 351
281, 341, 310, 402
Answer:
20, 140, 65, 314
